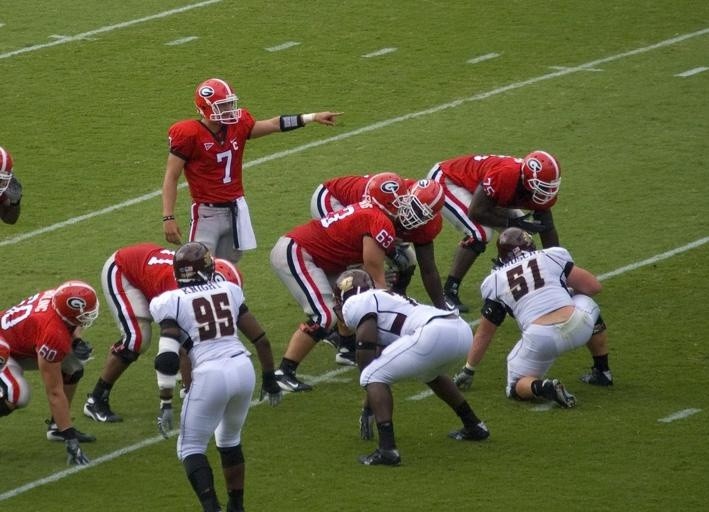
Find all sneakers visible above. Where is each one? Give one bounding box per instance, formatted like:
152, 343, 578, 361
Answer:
81, 393, 125, 423
273, 367, 312, 393
46, 418, 98, 446
581, 369, 616, 386
359, 448, 400, 467
447, 420, 491, 442
440, 283, 470, 313
541, 378, 579, 410
336, 348, 358, 368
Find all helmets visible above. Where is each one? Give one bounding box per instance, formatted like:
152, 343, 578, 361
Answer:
210, 255, 245, 293
51, 279, 99, 330
362, 172, 406, 220
519, 148, 563, 208
496, 226, 538, 263
332, 268, 374, 302
194, 78, 241, 126
396, 178, 447, 233
172, 242, 215, 288
0, 148, 12, 197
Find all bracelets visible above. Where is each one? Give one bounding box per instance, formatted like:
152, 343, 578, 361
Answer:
301, 112, 315, 125
280, 113, 304, 132
163, 215, 175, 221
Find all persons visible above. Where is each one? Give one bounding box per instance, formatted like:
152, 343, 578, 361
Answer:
426, 149, 563, 313
310, 176, 450, 348
163, 77, 344, 265
453, 227, 613, 407
150, 241, 283, 512
333, 268, 491, 466
84, 242, 244, 423
0, 280, 99, 465
269, 172, 412, 392
0, 146, 23, 224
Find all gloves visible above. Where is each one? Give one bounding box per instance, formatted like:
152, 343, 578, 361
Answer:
156, 400, 176, 440
65, 437, 90, 466
260, 372, 283, 409
451, 365, 477, 391
511, 211, 544, 235
71, 336, 94, 363
360, 408, 375, 441
